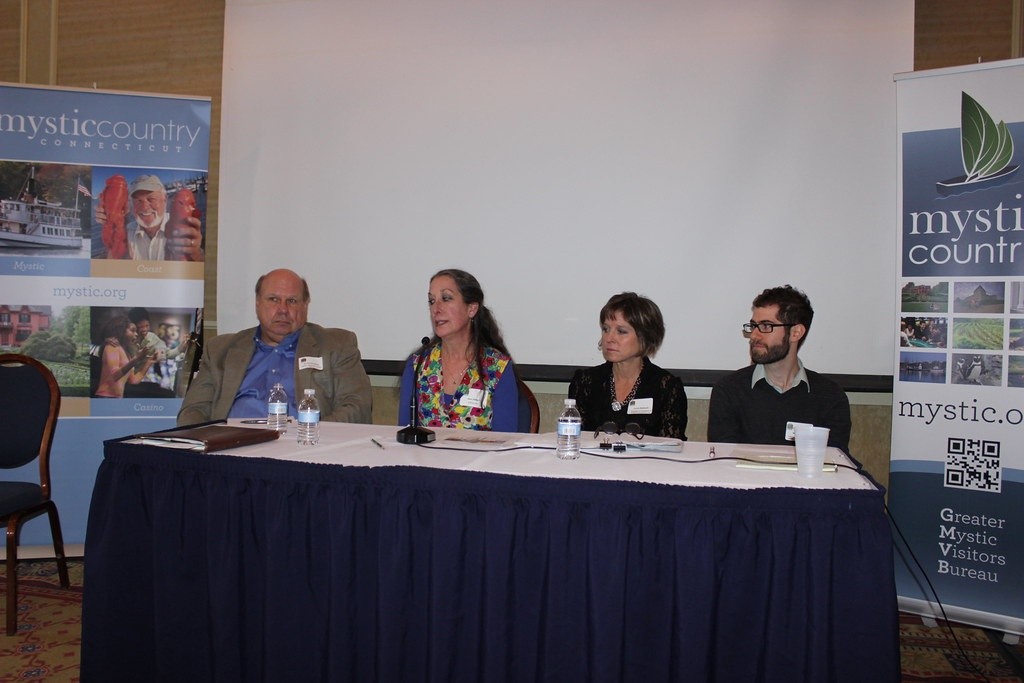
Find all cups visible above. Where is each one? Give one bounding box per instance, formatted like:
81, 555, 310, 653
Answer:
794, 425, 830, 479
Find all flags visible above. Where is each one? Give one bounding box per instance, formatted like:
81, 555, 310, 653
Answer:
78, 179, 91, 197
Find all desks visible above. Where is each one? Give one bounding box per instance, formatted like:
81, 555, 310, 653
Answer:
80, 416, 902, 683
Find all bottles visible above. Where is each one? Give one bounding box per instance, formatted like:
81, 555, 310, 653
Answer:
556, 399, 581, 461
267, 383, 288, 434
297, 389, 320, 445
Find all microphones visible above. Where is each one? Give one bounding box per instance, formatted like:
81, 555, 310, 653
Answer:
395, 336, 436, 445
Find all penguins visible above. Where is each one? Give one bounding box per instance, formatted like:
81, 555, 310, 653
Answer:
957, 355, 981, 384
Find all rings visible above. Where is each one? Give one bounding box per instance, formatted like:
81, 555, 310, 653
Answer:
191, 239, 194, 246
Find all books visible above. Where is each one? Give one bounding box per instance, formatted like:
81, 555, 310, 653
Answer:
140, 426, 279, 452
736, 452, 837, 471
513, 429, 684, 453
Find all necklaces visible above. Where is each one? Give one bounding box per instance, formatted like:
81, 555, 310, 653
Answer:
441, 350, 477, 385
609, 362, 646, 411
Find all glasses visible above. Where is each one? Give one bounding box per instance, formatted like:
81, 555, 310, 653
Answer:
594, 421, 645, 440
743, 322, 796, 333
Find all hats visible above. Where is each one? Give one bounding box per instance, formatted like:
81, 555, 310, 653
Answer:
129, 175, 160, 197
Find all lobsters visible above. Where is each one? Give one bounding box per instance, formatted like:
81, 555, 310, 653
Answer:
164, 189, 203, 261
98, 174, 130, 259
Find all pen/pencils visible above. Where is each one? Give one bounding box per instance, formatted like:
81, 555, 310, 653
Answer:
371, 439, 385, 450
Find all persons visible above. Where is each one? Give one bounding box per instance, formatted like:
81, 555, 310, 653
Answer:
398, 269, 518, 432
901, 320, 947, 348
568, 291, 688, 441
95, 173, 202, 261
95, 308, 193, 397
706, 287, 852, 454
178, 270, 372, 427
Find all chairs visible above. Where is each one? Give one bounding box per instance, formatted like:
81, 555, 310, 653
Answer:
0, 352, 71, 636
514, 377, 541, 434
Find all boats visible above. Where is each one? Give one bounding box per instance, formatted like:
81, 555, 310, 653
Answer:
0, 166, 83, 249
